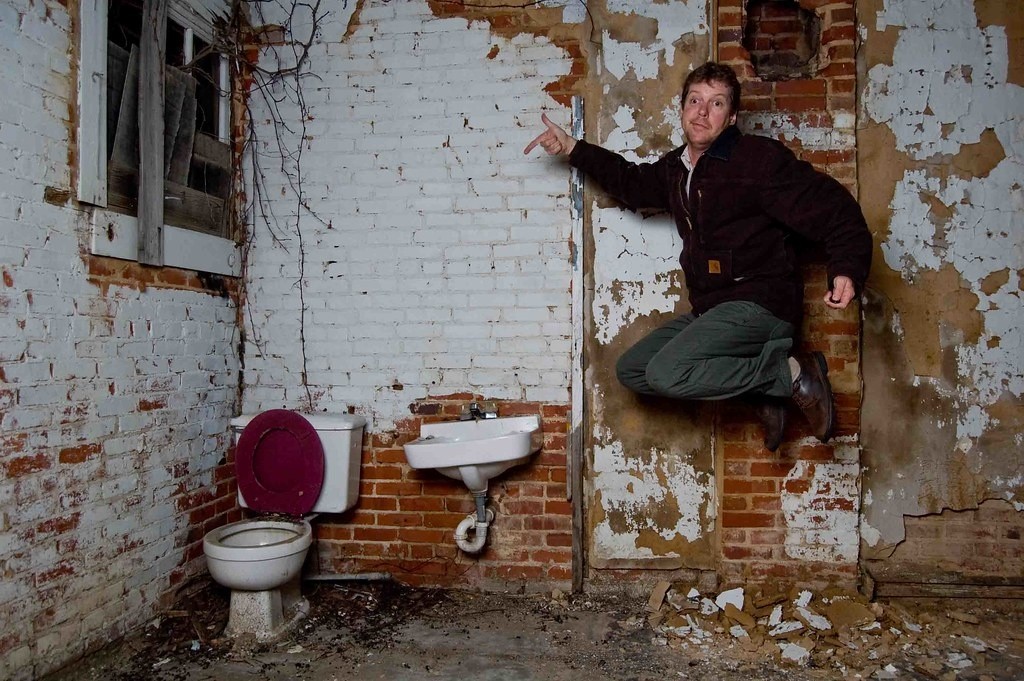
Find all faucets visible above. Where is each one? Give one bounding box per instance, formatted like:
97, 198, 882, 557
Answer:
469, 400, 488, 419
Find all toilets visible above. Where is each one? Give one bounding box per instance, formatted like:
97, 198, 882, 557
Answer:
202, 408, 366, 645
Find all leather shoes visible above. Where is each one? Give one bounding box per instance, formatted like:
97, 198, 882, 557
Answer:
748, 393, 788, 452
790, 350, 836, 445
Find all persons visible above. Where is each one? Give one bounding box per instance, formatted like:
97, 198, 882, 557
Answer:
523, 61, 873, 451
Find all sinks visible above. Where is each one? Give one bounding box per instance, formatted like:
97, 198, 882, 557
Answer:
402, 415, 545, 494
